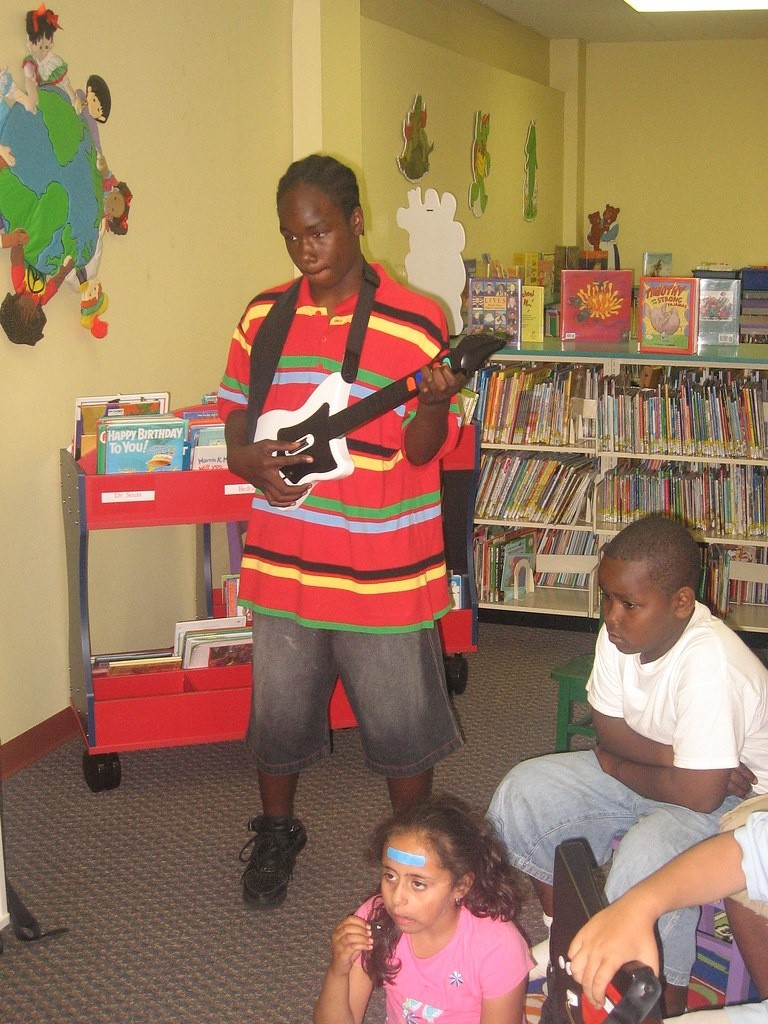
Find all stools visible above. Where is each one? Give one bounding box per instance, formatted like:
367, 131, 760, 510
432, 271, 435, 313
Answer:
694, 898, 751, 1006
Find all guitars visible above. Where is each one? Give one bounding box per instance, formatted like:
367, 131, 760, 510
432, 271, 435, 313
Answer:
248, 327, 511, 513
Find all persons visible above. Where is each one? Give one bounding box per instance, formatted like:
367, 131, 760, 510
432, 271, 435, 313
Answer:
472, 283, 517, 341
483, 516, 768, 1018
217, 154, 462, 910
315, 796, 535, 1024
566, 793, 768, 1024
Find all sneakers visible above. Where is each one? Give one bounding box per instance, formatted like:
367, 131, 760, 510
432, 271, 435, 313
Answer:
239, 817, 308, 909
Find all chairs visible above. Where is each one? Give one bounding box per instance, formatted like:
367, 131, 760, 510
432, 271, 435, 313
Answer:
536, 838, 662, 1023
551, 592, 604, 752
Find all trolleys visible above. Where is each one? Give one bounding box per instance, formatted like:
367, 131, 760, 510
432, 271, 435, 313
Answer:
57, 418, 483, 792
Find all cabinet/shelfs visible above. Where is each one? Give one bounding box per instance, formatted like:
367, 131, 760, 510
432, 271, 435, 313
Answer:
59, 418, 483, 791
449, 334, 768, 650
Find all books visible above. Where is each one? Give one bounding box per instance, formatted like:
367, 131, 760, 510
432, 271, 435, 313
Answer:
464, 256, 520, 278
89, 574, 253, 677
448, 570, 470, 611
469, 278, 521, 351
522, 286, 545, 343
474, 449, 768, 539
514, 245, 768, 347
473, 526, 768, 617
458, 362, 768, 460
637, 276, 699, 355
73, 393, 226, 473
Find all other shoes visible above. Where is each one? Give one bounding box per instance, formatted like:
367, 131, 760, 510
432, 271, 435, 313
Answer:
526, 937, 552, 982
